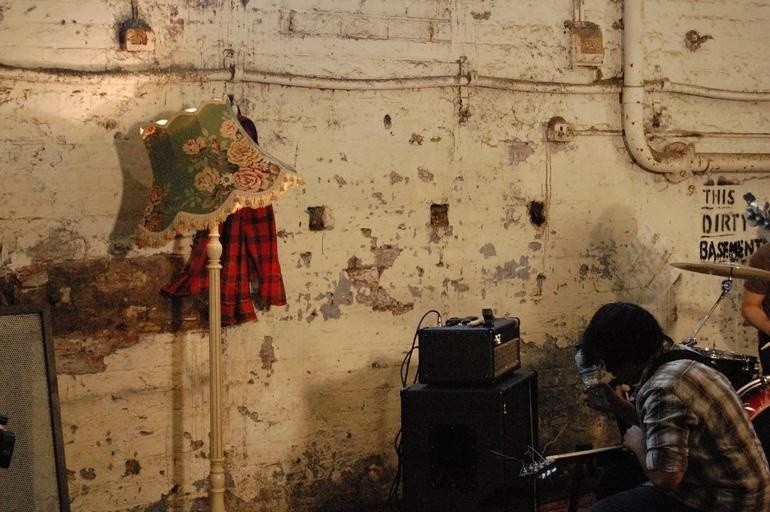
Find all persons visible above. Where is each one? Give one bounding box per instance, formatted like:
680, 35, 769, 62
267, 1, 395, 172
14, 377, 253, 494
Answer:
740, 241, 769, 377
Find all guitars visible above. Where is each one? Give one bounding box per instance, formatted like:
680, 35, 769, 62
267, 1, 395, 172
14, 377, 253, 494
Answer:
518, 445, 634, 480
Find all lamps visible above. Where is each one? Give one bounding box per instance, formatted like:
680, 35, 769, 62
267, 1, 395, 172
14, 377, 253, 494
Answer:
124, 93, 301, 510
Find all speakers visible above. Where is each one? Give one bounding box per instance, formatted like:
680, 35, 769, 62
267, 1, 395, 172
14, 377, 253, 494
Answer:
400, 369, 539, 512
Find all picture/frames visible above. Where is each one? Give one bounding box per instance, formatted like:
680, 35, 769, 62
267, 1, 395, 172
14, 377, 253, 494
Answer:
1, 302, 72, 512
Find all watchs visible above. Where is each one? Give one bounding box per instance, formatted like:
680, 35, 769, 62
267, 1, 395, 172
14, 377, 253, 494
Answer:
578, 298, 770, 510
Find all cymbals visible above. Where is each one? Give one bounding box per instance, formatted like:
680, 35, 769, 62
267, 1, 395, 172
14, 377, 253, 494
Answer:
669, 262, 770, 281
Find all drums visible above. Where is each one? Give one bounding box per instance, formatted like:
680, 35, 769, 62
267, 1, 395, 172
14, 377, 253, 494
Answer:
692, 347, 757, 392
736, 376, 770, 422
